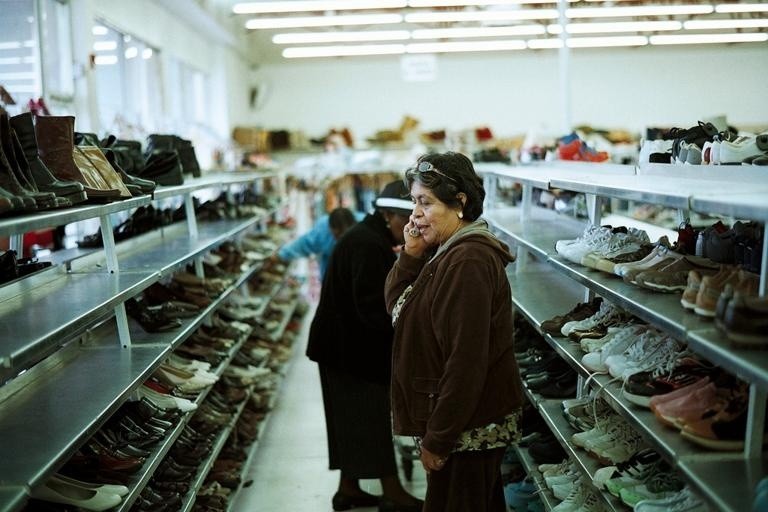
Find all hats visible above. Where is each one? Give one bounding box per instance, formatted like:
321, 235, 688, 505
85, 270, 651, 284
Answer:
371, 180, 415, 216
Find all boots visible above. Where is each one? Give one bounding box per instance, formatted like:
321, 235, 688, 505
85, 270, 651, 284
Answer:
1, 84, 200, 218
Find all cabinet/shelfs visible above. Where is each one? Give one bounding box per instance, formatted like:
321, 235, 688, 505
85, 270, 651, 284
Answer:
0, 171, 313, 512
473, 161, 768, 512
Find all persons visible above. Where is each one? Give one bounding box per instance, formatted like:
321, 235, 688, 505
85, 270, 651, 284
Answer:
384, 150, 527, 512
261, 206, 368, 286
305, 179, 425, 511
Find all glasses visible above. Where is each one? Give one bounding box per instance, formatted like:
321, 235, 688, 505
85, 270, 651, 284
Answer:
410, 159, 457, 187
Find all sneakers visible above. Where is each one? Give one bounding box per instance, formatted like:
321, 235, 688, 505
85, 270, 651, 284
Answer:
332, 491, 378, 511
524, 119, 768, 170
378, 487, 424, 512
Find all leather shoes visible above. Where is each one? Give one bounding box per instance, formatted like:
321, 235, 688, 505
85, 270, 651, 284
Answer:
472, 147, 510, 162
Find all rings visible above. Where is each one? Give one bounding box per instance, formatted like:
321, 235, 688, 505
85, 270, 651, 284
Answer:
435, 458, 445, 468
